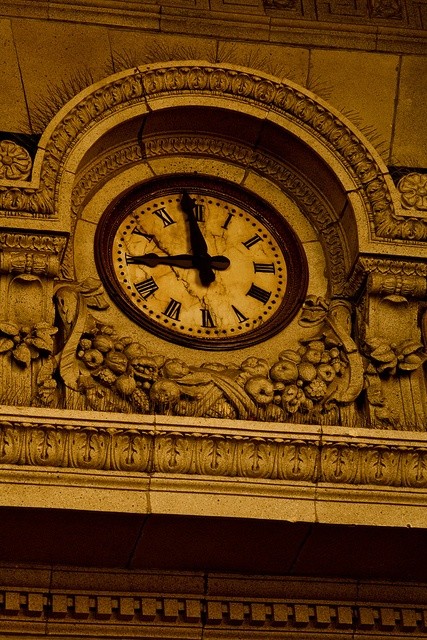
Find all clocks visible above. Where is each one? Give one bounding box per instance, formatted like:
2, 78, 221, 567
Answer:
93, 173, 310, 352
